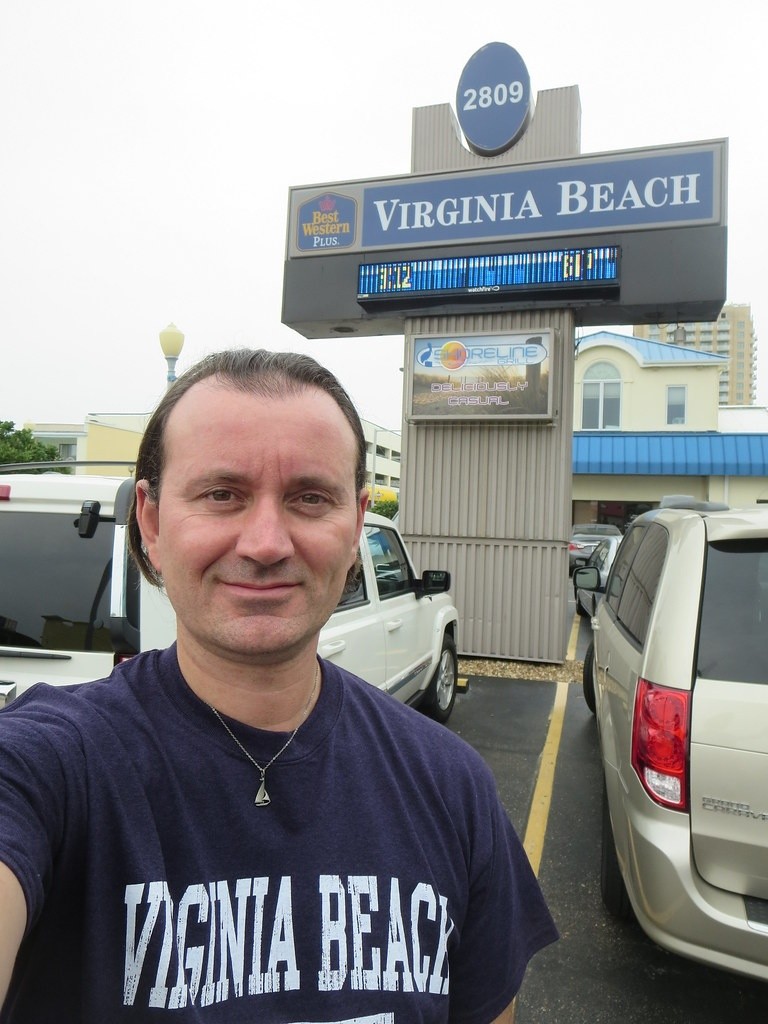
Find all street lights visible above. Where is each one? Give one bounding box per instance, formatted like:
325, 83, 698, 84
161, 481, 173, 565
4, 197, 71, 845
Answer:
159, 322, 184, 393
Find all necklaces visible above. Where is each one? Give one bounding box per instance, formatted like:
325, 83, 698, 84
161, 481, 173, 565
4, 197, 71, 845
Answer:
205, 663, 318, 806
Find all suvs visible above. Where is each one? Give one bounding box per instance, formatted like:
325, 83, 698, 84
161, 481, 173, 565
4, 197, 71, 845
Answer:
574, 502, 768, 985
0, 461, 460, 723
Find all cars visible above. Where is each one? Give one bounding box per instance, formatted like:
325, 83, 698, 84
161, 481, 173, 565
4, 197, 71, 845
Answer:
569, 523, 624, 576
575, 535, 624, 617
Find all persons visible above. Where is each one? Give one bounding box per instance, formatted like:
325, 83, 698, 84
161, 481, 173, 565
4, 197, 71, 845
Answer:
0, 350, 560, 1024
